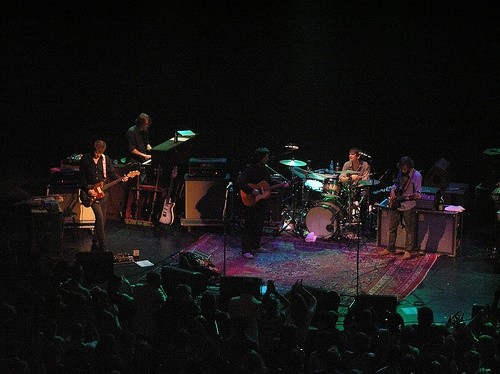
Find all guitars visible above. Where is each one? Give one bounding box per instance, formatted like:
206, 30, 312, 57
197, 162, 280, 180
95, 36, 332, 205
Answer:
158, 168, 178, 225
240, 176, 301, 207
79, 169, 141, 207
390, 189, 421, 209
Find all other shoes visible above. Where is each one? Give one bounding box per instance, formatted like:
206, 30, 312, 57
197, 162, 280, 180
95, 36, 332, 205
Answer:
378, 250, 396, 255
243, 253, 253, 259
100, 249, 111, 252
402, 252, 413, 261
91, 247, 98, 251
251, 246, 267, 253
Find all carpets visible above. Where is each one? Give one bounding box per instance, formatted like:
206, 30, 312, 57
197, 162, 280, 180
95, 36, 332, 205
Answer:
180, 228, 441, 303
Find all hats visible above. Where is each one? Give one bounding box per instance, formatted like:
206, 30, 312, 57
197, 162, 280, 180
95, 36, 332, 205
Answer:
358, 309, 372, 318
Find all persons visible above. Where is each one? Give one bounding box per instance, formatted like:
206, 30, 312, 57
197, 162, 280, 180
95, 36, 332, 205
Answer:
0, 273, 500, 374
339, 148, 370, 239
381, 156, 422, 259
237, 147, 290, 259
127, 112, 152, 188
81, 140, 128, 253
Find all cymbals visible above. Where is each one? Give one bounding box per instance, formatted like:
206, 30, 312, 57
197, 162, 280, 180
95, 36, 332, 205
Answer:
358, 179, 380, 185
335, 170, 361, 174
279, 159, 307, 166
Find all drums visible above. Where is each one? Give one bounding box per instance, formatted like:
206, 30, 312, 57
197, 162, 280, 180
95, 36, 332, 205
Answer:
305, 198, 347, 239
342, 180, 358, 195
321, 178, 341, 197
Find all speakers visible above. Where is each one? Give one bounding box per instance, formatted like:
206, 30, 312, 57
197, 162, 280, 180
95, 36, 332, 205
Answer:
44, 185, 97, 223
160, 265, 207, 299
219, 275, 262, 298
355, 294, 397, 318
183, 174, 233, 220
376, 199, 464, 258
292, 284, 340, 313
74, 252, 114, 283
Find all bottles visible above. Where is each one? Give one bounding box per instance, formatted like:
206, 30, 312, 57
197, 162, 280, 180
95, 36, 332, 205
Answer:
439, 194, 444, 210
336, 162, 340, 172
330, 160, 334, 174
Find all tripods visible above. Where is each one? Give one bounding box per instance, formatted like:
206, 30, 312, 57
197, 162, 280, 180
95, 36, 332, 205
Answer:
272, 166, 306, 243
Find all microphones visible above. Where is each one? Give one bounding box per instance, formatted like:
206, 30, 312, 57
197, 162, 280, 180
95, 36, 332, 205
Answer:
379, 171, 388, 180
359, 152, 371, 158
359, 196, 365, 203
285, 146, 299, 150
226, 182, 233, 189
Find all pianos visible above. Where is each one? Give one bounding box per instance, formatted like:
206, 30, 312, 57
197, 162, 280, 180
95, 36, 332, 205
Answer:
140, 130, 199, 221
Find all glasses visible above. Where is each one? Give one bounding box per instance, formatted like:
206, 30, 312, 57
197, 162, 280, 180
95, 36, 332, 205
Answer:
142, 122, 152, 126
